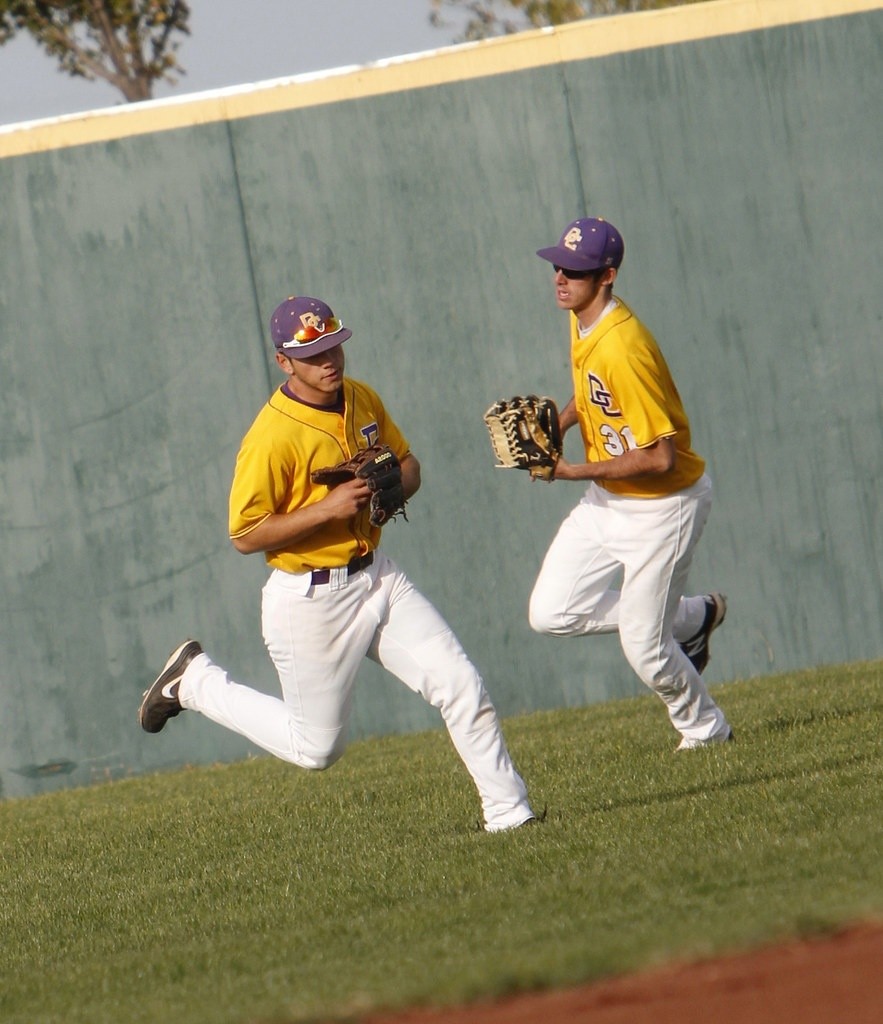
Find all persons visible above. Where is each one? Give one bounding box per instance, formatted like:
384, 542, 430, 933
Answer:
138, 297, 546, 833
527, 217, 737, 751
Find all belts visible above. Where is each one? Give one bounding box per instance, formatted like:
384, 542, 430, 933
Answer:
311, 550, 375, 585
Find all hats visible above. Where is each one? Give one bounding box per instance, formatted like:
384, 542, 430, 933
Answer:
270, 297, 352, 359
536, 218, 624, 269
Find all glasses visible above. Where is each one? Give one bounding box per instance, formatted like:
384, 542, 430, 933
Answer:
553, 264, 603, 280
277, 317, 344, 348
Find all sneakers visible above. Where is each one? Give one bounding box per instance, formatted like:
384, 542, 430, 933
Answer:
137, 638, 204, 733
680, 593, 728, 674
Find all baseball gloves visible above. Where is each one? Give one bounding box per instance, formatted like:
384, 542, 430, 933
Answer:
484, 392, 564, 485
310, 433, 410, 529
